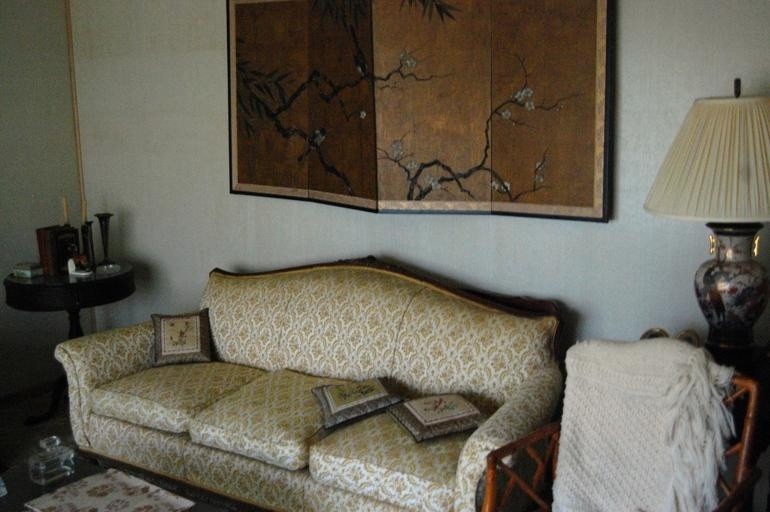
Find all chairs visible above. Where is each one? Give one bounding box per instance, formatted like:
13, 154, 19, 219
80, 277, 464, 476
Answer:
483, 339, 759, 511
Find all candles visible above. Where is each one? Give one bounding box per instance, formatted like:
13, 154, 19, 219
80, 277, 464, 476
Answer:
82, 200, 87, 225
62, 196, 69, 224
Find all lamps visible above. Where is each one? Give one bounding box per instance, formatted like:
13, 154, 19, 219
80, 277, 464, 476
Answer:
642, 93, 770, 353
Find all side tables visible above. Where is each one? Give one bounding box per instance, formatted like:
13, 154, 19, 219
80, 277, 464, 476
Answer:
3, 262, 137, 425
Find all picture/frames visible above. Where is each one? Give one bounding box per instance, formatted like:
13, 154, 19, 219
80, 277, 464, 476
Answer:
225, 0, 615, 223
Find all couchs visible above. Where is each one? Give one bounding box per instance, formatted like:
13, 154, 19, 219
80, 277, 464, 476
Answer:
53, 255, 565, 512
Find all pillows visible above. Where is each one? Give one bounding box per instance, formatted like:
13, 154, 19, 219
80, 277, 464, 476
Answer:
150, 307, 214, 366
309, 377, 489, 445
